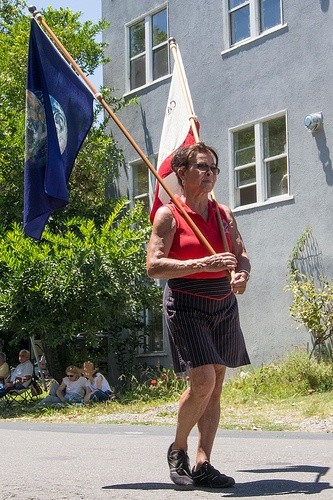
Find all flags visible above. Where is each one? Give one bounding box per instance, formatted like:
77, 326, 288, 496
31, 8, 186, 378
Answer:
24, 19, 95, 240
150, 45, 200, 226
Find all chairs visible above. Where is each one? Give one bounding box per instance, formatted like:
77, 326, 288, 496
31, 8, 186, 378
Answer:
0, 359, 43, 409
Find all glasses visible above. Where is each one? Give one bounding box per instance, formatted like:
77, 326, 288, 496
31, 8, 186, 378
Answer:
189, 163, 220, 175
66, 375, 73, 377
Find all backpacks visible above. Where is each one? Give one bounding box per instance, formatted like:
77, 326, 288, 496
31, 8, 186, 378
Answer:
47, 378, 64, 396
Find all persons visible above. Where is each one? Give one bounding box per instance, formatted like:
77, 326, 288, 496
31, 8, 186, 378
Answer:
0, 349, 33, 398
22, 365, 91, 413
0, 352, 9, 384
146, 142, 251, 488
80, 361, 112, 405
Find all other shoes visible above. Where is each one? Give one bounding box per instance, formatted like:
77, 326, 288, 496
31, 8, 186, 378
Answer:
22, 407, 34, 412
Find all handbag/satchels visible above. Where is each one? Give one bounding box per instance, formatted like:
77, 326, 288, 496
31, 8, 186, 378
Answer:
30, 380, 42, 396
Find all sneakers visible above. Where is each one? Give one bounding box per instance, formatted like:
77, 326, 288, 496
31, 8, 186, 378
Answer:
190, 461, 235, 488
167, 442, 196, 486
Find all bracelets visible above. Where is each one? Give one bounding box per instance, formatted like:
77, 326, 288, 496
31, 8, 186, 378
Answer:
239, 270, 250, 280
